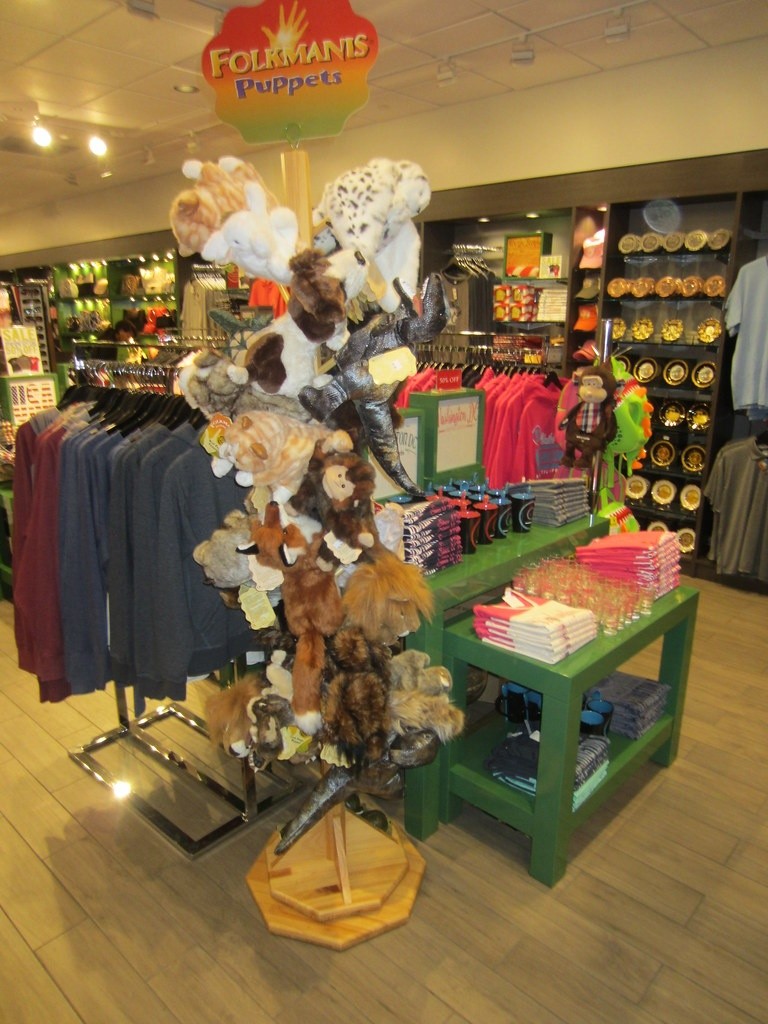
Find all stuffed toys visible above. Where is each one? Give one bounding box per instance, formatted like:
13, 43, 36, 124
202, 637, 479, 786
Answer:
553, 353, 654, 490
170, 154, 466, 856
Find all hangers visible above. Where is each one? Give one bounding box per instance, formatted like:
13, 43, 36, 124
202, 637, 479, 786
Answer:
442, 243, 488, 274
188, 264, 222, 284
56, 358, 207, 437
416, 342, 564, 391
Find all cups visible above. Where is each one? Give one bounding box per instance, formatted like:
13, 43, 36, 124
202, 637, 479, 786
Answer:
513, 554, 653, 636
496, 681, 615, 736
387, 480, 535, 554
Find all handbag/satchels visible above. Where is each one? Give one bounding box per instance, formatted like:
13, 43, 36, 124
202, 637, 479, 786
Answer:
59, 271, 109, 299
66, 310, 108, 332
121, 276, 140, 296
122, 309, 177, 334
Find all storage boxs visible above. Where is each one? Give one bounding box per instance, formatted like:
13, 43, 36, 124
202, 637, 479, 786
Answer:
511, 285, 535, 305
492, 303, 512, 322
503, 231, 554, 280
491, 285, 513, 303
509, 302, 532, 322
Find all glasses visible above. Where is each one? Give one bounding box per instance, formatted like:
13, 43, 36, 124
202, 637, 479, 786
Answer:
42, 361, 47, 365
37, 334, 44, 340
24, 307, 40, 315
39, 343, 45, 348
23, 299, 40, 304
21, 288, 39, 295
44, 370, 48, 373
25, 316, 42, 323
41, 352, 46, 355
37, 326, 43, 331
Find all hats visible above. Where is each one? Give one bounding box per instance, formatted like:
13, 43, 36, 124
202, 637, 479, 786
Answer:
579, 236, 604, 268
574, 276, 600, 299
573, 303, 599, 332
572, 339, 597, 362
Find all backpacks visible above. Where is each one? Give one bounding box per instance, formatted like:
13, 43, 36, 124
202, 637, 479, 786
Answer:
602, 357, 654, 453
554, 372, 582, 458
561, 363, 618, 468
596, 488, 640, 536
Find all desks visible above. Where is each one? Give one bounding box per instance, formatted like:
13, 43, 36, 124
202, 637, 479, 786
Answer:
218, 513, 609, 842
438, 584, 701, 891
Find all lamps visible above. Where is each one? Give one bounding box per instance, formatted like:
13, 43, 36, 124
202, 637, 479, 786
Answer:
604, 7, 631, 44
509, 34, 534, 65
437, 57, 459, 87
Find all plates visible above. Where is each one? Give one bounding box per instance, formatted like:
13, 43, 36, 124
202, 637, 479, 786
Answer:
604, 227, 732, 554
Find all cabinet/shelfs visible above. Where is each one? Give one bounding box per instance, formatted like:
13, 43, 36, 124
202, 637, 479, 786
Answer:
0, 146, 768, 598
